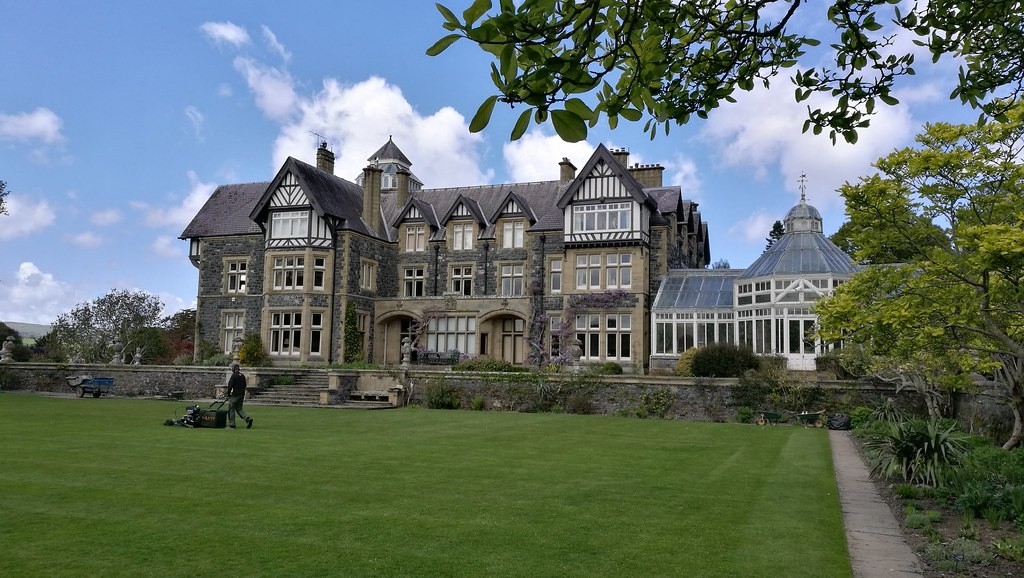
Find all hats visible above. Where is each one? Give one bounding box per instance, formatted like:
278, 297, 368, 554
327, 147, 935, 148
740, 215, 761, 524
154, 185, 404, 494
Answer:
232, 364, 240, 372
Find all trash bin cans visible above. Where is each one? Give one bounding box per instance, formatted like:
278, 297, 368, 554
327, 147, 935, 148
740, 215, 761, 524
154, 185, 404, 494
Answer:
387, 387, 405, 406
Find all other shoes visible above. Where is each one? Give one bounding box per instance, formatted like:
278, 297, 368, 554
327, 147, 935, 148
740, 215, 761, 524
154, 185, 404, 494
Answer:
226, 425, 236, 429
247, 419, 253, 428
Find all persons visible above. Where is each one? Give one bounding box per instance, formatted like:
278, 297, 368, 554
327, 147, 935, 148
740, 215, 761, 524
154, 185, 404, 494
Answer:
225, 363, 253, 429
